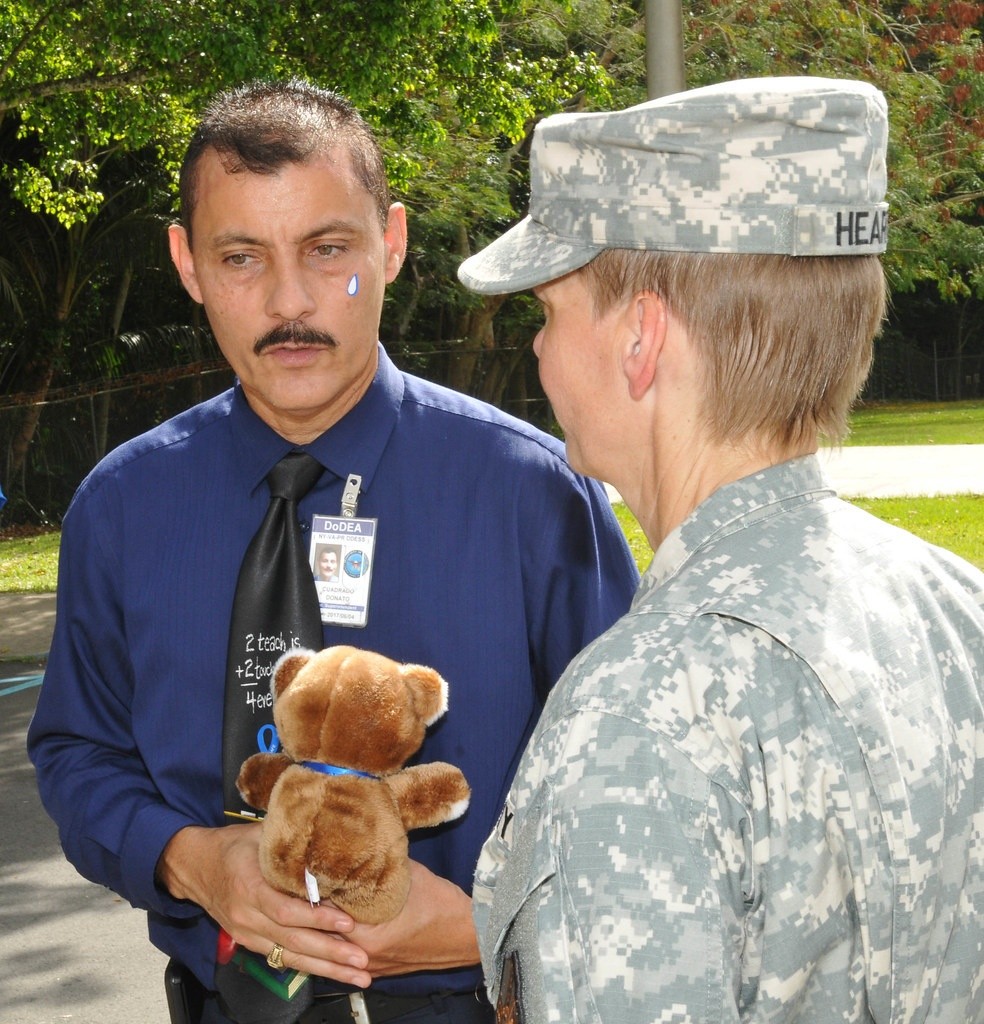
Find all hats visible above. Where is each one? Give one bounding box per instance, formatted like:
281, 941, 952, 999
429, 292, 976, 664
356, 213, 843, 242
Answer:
456, 73, 892, 296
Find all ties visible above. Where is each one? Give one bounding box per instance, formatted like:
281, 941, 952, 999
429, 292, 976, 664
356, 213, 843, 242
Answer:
211, 454, 314, 1023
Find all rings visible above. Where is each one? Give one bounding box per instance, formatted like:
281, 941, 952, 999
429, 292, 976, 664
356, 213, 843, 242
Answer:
267, 943, 286, 970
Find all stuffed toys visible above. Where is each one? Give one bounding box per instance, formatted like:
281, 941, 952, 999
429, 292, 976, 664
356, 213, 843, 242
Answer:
235, 645, 472, 925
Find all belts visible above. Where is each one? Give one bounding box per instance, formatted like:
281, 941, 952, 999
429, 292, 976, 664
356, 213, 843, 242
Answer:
296, 989, 456, 1024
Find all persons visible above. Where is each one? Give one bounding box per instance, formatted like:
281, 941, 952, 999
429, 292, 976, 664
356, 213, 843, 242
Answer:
24, 76, 642, 1024
453, 72, 984, 1024
